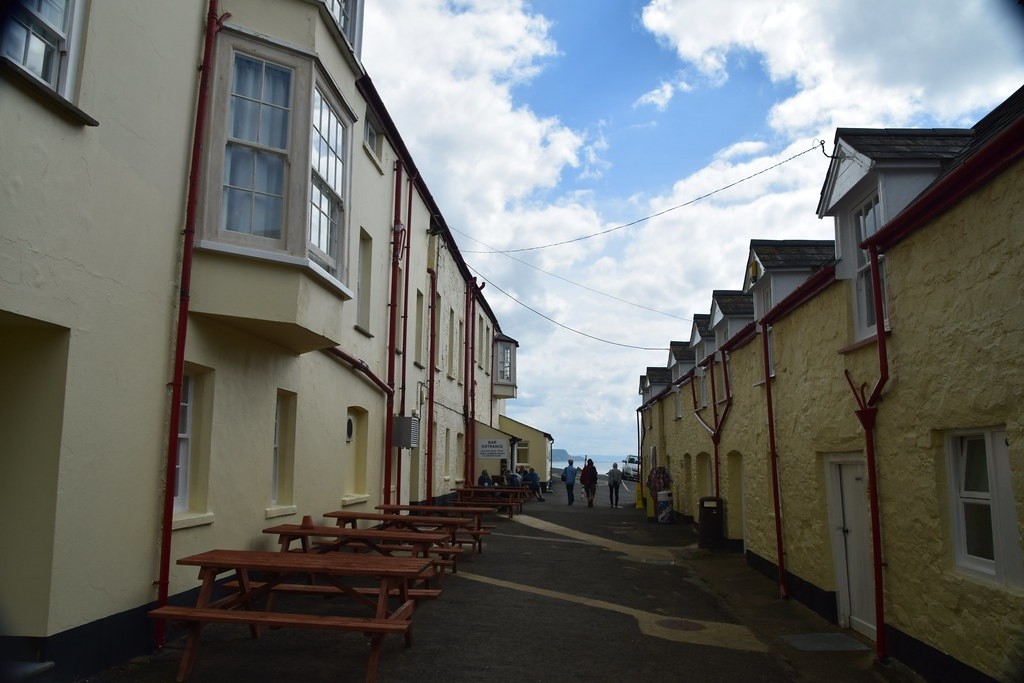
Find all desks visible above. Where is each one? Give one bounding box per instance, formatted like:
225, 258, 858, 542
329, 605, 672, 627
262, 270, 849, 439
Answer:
373, 503, 497, 554
444, 483, 532, 520
147, 548, 443, 683
320, 510, 478, 573
261, 523, 465, 600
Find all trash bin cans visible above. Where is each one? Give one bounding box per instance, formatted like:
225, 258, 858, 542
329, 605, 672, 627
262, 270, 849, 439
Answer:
656, 490, 673, 525
698, 495, 726, 551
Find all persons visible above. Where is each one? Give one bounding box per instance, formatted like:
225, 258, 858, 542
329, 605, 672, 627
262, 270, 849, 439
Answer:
478, 469, 492, 486
504, 470, 522, 486
518, 466, 528, 475
561, 460, 577, 505
523, 469, 545, 502
608, 463, 622, 507
580, 459, 597, 507
